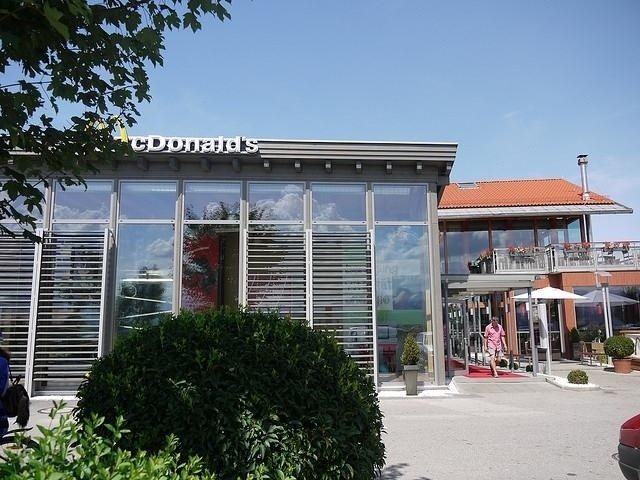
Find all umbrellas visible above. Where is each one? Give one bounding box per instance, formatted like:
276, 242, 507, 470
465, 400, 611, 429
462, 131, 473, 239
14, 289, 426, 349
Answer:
510, 285, 589, 349
574, 290, 640, 307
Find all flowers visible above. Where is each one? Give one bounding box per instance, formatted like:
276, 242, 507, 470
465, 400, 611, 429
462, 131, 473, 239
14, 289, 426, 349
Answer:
478, 248, 494, 259
508, 239, 630, 256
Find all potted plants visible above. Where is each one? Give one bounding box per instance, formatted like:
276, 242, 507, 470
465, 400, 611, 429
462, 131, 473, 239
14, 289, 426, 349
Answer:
399, 332, 421, 395
570, 324, 601, 361
603, 336, 635, 374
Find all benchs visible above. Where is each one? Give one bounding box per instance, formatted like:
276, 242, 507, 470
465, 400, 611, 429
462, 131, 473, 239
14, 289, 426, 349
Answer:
580, 352, 607, 367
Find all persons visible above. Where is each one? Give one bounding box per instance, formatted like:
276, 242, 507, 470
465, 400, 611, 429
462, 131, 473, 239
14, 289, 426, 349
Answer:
0, 347, 13, 437
483, 316, 509, 378
